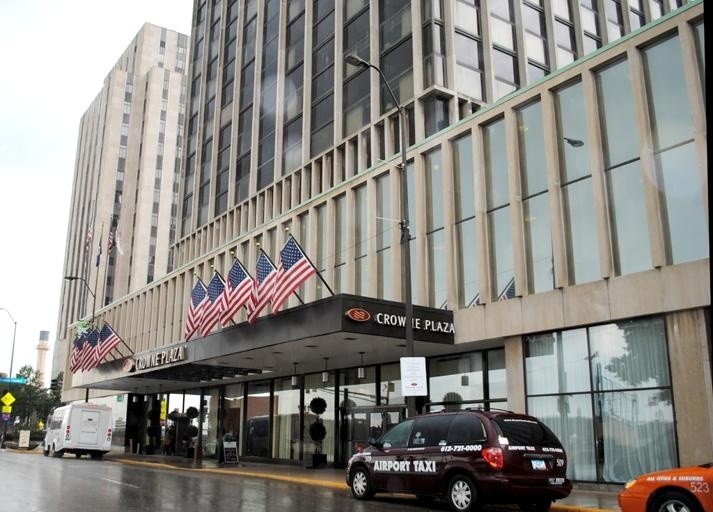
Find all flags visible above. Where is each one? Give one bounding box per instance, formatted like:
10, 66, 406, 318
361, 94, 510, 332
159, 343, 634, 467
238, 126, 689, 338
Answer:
94, 236, 103, 267
67, 321, 122, 374
197, 272, 225, 338
182, 281, 207, 343
245, 251, 275, 324
84, 226, 91, 256
106, 231, 115, 252
270, 238, 317, 321
217, 261, 251, 329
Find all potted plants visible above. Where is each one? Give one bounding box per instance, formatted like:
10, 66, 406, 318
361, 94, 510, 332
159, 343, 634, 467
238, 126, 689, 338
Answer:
301, 396, 328, 469
181, 406, 198, 458
142, 409, 158, 455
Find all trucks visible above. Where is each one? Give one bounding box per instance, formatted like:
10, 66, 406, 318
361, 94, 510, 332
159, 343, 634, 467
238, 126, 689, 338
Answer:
43, 400, 114, 460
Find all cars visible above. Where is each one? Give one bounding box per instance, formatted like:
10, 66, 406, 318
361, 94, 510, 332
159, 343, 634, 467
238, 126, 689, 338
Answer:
618, 462, 713, 512
221, 411, 368, 464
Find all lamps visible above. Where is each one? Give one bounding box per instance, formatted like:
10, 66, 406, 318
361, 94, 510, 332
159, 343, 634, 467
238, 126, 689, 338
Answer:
356, 351, 366, 379
290, 361, 299, 388
320, 356, 330, 383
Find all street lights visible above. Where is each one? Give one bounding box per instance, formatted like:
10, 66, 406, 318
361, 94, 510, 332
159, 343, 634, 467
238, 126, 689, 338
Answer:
0, 309, 17, 449
64, 275, 97, 402
336, 49, 423, 421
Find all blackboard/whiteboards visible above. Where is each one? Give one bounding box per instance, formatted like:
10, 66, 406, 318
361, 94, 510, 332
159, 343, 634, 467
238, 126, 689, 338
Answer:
224, 447, 239, 464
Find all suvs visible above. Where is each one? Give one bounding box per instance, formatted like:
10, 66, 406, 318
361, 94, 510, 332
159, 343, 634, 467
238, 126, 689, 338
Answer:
346, 407, 573, 510
